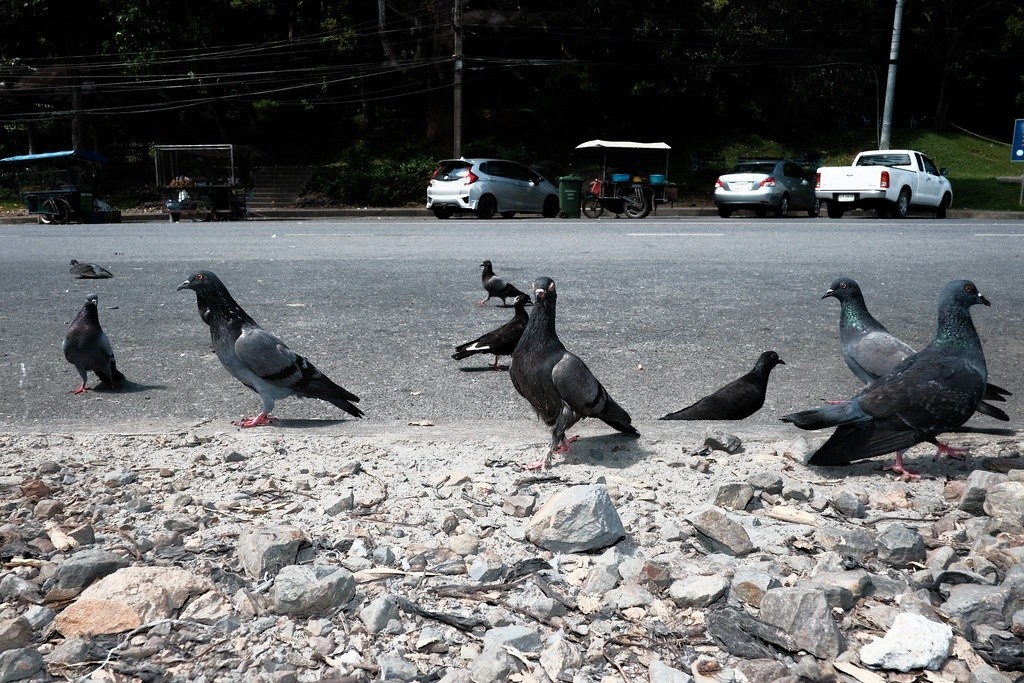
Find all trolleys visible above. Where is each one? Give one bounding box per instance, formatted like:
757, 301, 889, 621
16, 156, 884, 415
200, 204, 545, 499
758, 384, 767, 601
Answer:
154, 143, 255, 223
0, 149, 109, 225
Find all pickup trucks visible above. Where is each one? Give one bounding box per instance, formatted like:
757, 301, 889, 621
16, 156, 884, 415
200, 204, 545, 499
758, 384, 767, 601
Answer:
812, 148, 955, 219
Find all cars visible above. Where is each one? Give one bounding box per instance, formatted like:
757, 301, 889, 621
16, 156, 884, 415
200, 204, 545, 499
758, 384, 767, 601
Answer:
424, 155, 560, 220
713, 158, 823, 218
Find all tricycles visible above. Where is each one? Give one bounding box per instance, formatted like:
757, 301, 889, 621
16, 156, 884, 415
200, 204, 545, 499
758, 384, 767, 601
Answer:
573, 139, 677, 219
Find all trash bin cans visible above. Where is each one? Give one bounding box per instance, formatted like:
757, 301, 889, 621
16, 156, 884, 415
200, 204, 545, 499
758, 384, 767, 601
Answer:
556, 176, 584, 219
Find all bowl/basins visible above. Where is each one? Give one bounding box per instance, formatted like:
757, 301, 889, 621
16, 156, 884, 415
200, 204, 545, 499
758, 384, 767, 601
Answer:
612, 174, 631, 182
650, 174, 664, 183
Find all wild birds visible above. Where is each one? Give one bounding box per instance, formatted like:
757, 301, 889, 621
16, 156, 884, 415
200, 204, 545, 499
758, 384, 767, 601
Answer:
782, 274, 1012, 479
63, 294, 128, 396
177, 270, 365, 428
458, 256, 639, 458
658, 351, 787, 419
69, 257, 113, 280
